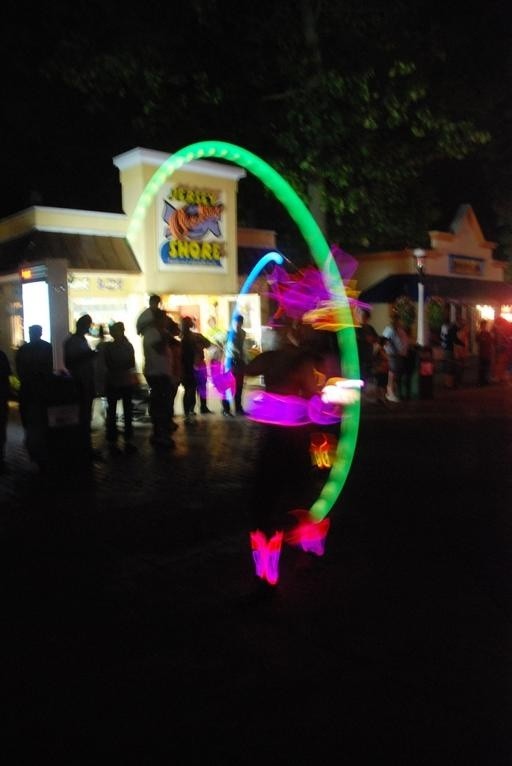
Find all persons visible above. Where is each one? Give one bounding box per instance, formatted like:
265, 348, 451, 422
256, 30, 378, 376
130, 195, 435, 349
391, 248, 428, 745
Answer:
355, 306, 410, 403
16, 291, 265, 469
474, 319, 497, 389
438, 314, 466, 389
1, 348, 14, 467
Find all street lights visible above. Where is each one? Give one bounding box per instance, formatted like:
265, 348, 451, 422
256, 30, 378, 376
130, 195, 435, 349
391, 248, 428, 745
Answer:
406, 244, 439, 397
